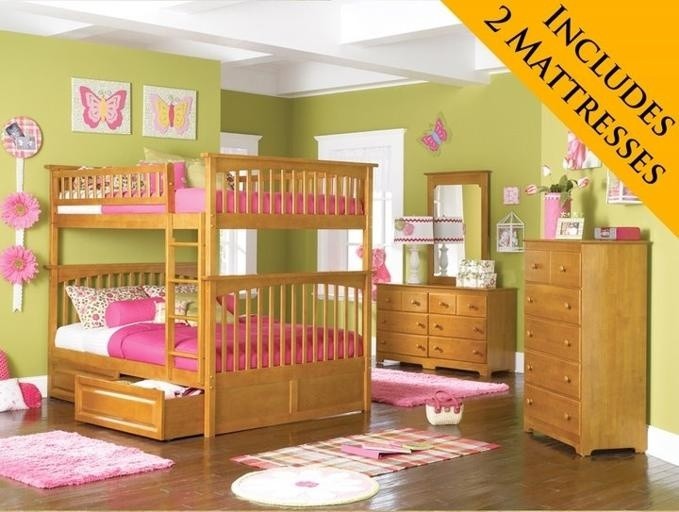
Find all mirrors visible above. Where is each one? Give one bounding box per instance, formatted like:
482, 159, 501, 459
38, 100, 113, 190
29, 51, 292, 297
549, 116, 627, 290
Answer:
434, 184, 481, 277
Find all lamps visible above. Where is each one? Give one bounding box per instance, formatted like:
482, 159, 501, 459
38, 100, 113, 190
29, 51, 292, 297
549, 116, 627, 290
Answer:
393, 213, 434, 284
434, 214, 464, 276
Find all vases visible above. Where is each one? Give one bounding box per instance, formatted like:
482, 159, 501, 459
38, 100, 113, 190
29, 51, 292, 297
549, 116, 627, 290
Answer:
544, 193, 570, 239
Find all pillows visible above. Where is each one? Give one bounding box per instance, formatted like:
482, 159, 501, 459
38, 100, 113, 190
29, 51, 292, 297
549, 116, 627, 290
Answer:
0, 378, 42, 412
66, 285, 276, 328
74, 146, 234, 192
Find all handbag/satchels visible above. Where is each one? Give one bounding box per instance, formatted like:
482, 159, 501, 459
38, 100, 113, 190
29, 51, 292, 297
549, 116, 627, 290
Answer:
426, 390, 464, 425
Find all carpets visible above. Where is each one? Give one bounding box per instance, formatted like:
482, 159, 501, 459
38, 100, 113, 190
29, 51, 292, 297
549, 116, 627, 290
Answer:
0, 431, 175, 489
371, 367, 509, 406
229, 427, 501, 508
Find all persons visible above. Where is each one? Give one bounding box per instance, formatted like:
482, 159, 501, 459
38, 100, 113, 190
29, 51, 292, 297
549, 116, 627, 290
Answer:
565, 223, 577, 235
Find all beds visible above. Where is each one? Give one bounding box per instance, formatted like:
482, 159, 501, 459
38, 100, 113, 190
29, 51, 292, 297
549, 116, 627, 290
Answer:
44, 152, 378, 439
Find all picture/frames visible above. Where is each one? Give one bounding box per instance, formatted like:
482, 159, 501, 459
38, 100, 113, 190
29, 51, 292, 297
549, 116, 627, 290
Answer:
556, 218, 585, 240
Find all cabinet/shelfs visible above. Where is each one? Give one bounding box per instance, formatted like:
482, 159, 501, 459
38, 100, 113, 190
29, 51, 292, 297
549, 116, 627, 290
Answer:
522, 240, 650, 457
374, 283, 518, 376
48, 357, 118, 409
74, 374, 205, 440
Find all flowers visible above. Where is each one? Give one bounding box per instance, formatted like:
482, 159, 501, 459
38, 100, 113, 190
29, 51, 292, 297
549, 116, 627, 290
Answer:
0, 245, 39, 283
524, 165, 590, 195
2, 192, 41, 230
